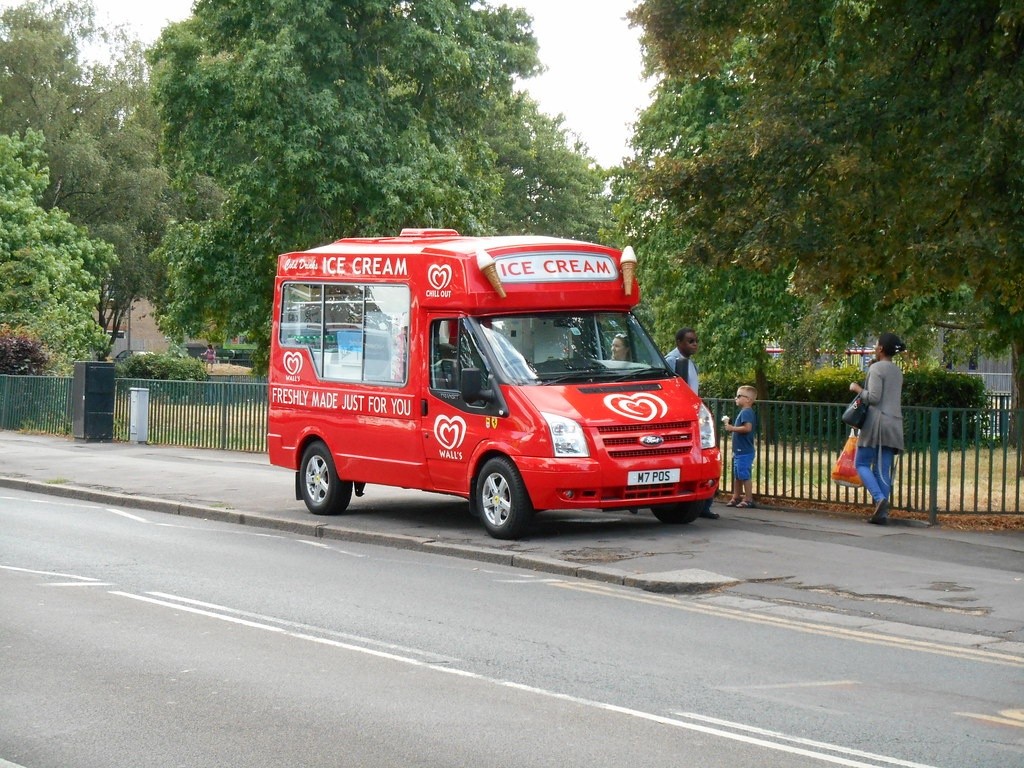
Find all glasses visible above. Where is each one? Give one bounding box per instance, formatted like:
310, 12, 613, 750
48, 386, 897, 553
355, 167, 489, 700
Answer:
736, 395, 749, 398
683, 339, 699, 344
615, 334, 628, 339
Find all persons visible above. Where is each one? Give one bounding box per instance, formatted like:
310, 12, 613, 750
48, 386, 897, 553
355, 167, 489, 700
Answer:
629, 328, 718, 520
200, 345, 215, 372
609, 334, 631, 361
850, 332, 907, 526
724, 385, 758, 509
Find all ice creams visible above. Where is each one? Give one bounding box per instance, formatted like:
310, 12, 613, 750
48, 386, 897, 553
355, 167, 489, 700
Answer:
620, 245, 637, 295
722, 416, 730, 426
476, 248, 506, 298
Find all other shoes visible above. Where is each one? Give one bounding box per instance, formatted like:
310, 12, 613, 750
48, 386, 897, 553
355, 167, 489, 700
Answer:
873, 497, 887, 517
699, 510, 719, 519
868, 516, 887, 525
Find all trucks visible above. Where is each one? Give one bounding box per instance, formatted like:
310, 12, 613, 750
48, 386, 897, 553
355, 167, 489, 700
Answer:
266, 228, 723, 540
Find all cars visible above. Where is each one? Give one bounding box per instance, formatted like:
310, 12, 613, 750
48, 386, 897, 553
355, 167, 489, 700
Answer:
111, 350, 154, 363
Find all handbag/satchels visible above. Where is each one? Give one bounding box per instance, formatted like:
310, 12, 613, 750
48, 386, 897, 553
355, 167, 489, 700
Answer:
842, 391, 870, 430
832, 428, 864, 487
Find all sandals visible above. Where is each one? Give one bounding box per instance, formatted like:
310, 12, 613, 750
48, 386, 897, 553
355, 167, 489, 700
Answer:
736, 498, 754, 508
726, 498, 741, 506
